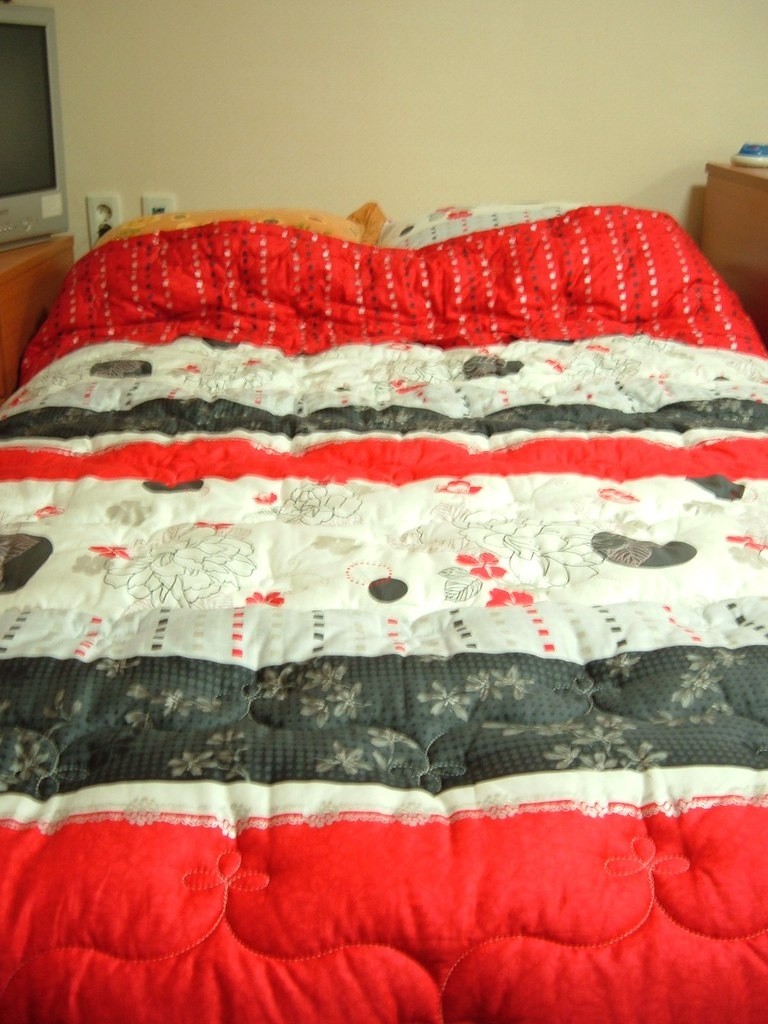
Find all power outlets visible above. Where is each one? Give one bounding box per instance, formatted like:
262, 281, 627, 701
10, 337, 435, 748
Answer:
86, 195, 122, 250
140, 195, 176, 217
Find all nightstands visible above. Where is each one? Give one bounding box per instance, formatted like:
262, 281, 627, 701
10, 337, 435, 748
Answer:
698, 162, 768, 353
0, 234, 76, 401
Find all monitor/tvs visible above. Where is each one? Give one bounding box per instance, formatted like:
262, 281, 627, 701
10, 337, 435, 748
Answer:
0, 4, 69, 254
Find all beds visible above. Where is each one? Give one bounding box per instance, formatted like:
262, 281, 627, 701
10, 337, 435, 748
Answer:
1, 205, 768, 1023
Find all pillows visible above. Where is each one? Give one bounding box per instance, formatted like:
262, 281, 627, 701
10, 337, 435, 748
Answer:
378, 205, 591, 251
88, 199, 389, 252
